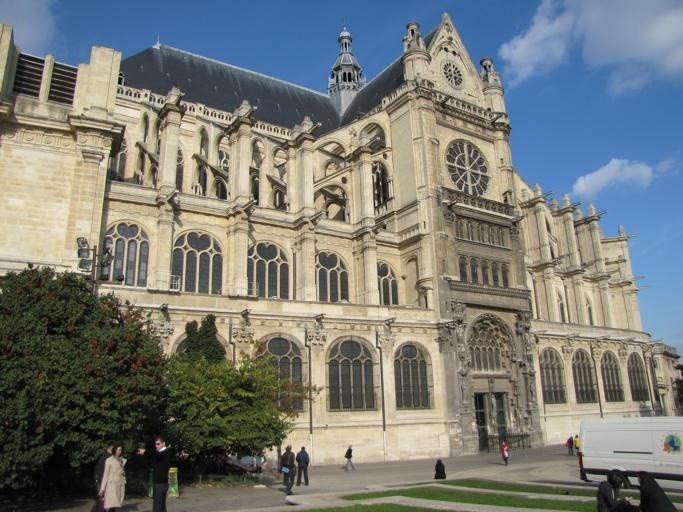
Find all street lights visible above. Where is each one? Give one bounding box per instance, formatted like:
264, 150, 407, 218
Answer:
74, 236, 113, 296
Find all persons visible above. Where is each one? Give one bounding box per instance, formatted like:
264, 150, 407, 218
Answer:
280, 445, 297, 495
295, 446, 310, 486
499, 440, 510, 466
93, 434, 176, 512
434, 460, 447, 479
565, 433, 680, 512
344, 444, 356, 471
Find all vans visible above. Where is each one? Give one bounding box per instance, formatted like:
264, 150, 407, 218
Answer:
578, 416, 682, 495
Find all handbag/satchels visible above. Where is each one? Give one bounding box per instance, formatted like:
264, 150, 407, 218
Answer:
282, 466, 290, 474
90, 495, 105, 512
